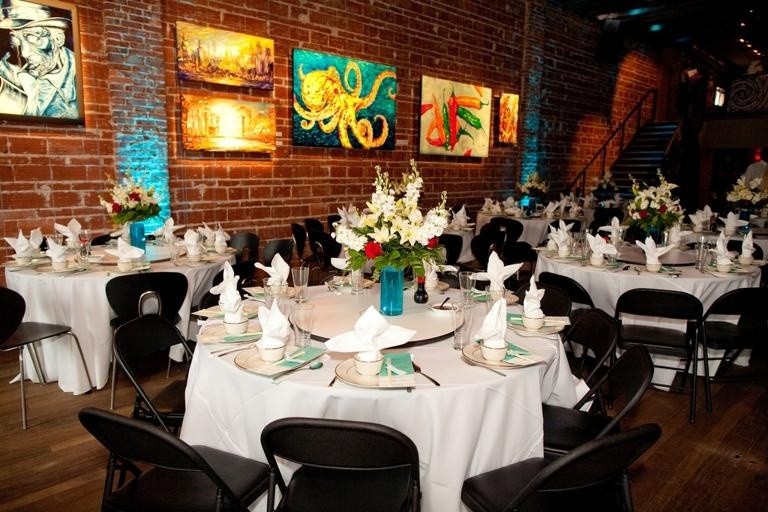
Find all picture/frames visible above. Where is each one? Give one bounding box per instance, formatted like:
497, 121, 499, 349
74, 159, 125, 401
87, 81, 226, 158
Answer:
1, 0, 92, 135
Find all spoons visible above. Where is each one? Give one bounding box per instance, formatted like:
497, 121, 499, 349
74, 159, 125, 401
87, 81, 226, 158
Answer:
272, 361, 324, 380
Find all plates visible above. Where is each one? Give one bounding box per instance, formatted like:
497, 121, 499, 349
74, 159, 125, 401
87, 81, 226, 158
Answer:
432, 303, 459, 316
510, 318, 566, 337
4, 224, 238, 276
202, 295, 268, 361
318, 257, 521, 306
234, 346, 300, 372
535, 222, 762, 279
333, 354, 382, 389
461, 341, 520, 372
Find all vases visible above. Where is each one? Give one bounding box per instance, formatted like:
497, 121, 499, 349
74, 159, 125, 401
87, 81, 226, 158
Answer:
124, 215, 150, 259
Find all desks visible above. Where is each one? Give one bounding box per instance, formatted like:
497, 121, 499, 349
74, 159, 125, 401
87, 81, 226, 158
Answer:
472, 202, 597, 251
527, 238, 761, 391
180, 280, 594, 510
1, 241, 238, 387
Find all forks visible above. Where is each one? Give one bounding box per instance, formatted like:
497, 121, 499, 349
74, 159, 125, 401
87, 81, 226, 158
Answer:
409, 359, 443, 389
458, 354, 509, 379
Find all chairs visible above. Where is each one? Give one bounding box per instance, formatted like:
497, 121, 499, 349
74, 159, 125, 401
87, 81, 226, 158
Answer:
558, 306, 623, 382
105, 270, 194, 411
543, 343, 658, 509
259, 416, 423, 511
678, 284, 768, 387
112, 312, 196, 435
76, 405, 270, 512
1, 286, 97, 429
458, 421, 667, 512
85, 198, 768, 324
613, 287, 704, 426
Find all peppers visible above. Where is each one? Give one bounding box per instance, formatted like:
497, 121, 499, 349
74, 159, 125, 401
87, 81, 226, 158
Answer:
421, 85, 489, 157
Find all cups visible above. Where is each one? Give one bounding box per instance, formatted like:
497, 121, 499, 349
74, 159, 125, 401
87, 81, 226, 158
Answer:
451, 305, 474, 351
291, 266, 309, 304
290, 302, 315, 348
261, 276, 289, 315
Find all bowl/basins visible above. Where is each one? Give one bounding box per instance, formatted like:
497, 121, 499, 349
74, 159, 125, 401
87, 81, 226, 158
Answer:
520, 313, 544, 329
352, 350, 385, 375
479, 337, 509, 362
253, 338, 286, 363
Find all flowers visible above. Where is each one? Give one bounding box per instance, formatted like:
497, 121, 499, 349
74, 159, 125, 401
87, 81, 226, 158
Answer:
328, 157, 455, 280
515, 173, 552, 197
97, 182, 162, 222
624, 167, 687, 233
725, 177, 764, 207
